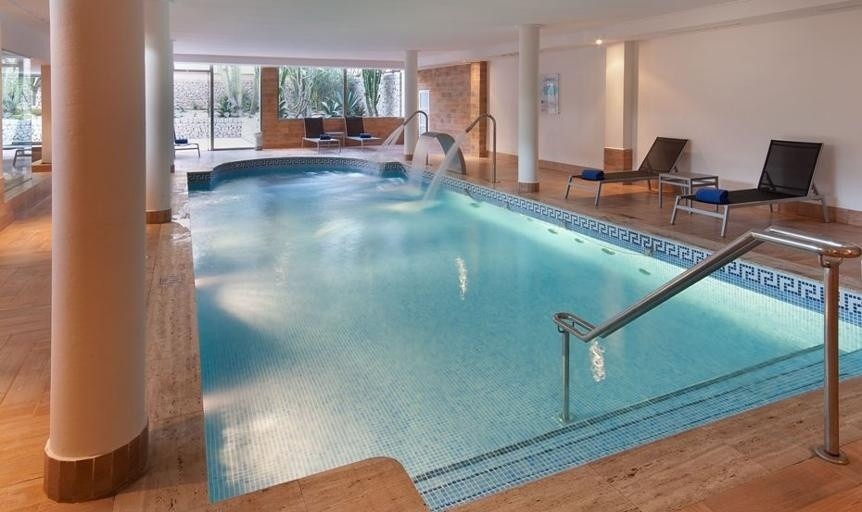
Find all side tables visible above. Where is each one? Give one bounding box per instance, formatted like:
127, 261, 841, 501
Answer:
659, 171, 719, 214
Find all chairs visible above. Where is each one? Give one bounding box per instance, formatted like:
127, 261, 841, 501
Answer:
344, 116, 383, 152
565, 137, 689, 207
13, 148, 32, 167
301, 117, 342, 153
670, 139, 829, 237
174, 131, 200, 159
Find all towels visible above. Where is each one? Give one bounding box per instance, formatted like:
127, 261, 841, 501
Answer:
320, 134, 332, 141
359, 133, 372, 138
696, 186, 729, 204
580, 169, 605, 181
175, 139, 187, 145
